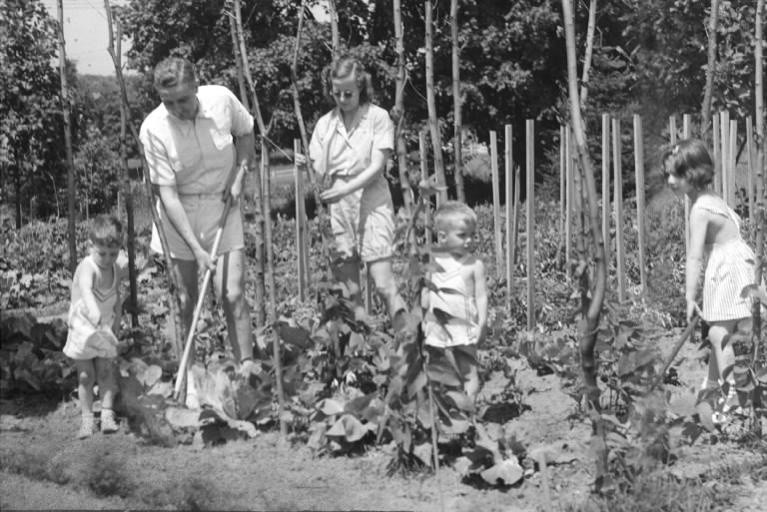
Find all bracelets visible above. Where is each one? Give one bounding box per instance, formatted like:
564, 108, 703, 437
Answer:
234, 162, 249, 170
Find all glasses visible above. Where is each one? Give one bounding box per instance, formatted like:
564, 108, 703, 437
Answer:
328, 90, 353, 97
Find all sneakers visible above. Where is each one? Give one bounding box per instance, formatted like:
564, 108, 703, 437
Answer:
100, 413, 118, 433
78, 420, 93, 439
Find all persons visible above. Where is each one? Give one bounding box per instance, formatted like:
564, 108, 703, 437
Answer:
294, 57, 410, 341
138, 53, 260, 387
660, 135, 760, 402
62, 212, 132, 440
420, 198, 491, 363
